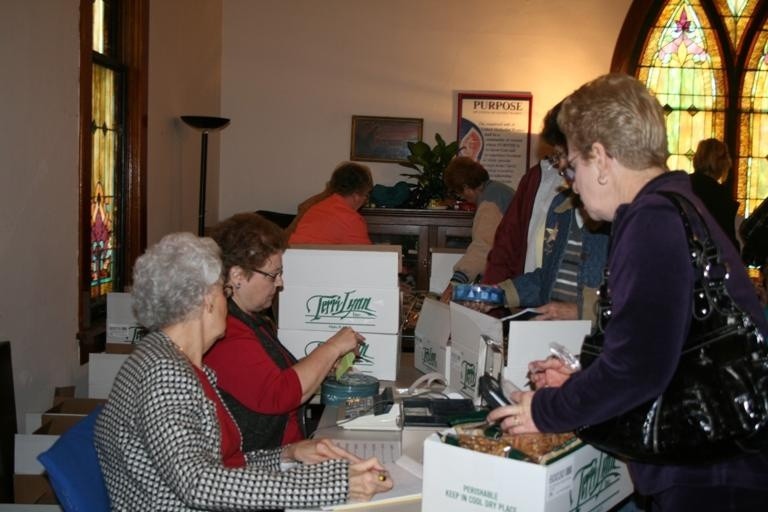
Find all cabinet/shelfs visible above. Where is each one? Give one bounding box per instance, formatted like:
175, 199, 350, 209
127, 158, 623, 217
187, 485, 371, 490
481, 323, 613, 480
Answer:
359, 211, 480, 290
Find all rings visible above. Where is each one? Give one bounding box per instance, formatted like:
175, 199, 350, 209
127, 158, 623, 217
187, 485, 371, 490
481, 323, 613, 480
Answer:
513, 415, 522, 426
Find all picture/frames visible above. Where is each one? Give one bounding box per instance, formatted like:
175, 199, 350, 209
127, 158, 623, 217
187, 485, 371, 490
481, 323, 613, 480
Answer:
455, 92, 533, 191
350, 115, 423, 164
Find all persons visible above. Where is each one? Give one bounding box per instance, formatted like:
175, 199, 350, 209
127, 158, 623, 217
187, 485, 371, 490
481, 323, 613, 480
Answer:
91, 229, 396, 512
483, 73, 768, 512
201, 213, 373, 446
441, 106, 768, 327
289, 162, 374, 246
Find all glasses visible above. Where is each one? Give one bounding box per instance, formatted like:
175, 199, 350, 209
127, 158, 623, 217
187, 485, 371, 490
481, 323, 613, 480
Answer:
559, 154, 580, 180
251, 266, 277, 281
217, 284, 233, 298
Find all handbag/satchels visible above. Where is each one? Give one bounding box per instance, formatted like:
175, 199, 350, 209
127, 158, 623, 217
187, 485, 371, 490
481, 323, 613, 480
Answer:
573, 190, 767, 463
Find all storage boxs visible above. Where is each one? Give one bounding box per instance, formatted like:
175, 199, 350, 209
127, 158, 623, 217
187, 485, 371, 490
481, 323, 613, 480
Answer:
14, 285, 148, 503
